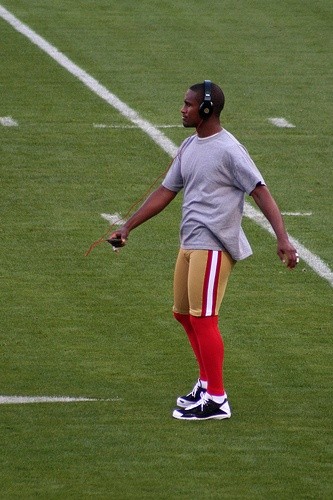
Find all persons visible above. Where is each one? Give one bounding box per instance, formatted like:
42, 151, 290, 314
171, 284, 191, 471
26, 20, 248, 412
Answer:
109, 83, 301, 420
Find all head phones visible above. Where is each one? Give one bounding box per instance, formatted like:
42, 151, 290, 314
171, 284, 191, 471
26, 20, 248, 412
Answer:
199, 79, 214, 121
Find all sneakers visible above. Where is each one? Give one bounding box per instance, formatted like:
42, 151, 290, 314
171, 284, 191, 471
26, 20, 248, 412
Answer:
176, 380, 208, 408
174, 393, 231, 421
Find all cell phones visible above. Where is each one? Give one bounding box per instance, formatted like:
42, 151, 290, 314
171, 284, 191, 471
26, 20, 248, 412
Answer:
107, 239, 124, 247
